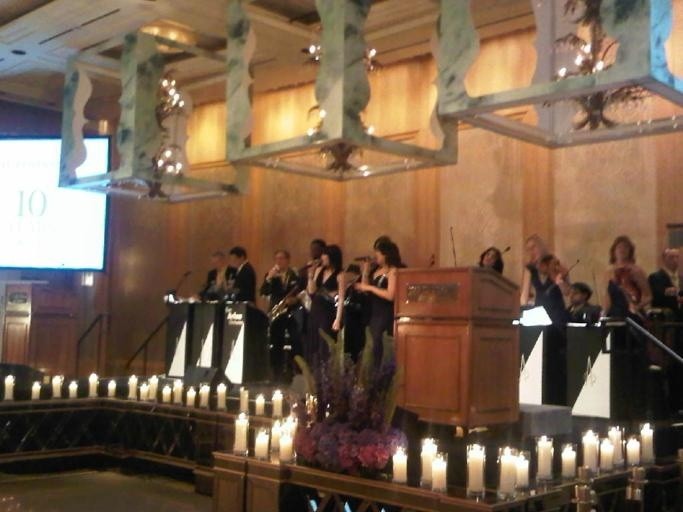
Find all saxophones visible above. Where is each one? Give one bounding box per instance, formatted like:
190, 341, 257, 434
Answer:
267, 281, 301, 323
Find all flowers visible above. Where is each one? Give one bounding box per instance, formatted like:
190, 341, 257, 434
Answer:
295, 325, 408, 475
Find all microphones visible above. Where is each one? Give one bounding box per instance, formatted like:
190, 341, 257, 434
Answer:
354, 255, 376, 260
298, 259, 322, 273
450, 226, 457, 267
487, 247, 511, 267
175, 270, 190, 292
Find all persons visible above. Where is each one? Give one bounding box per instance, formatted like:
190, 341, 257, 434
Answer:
479, 247, 505, 273
600, 237, 653, 370
519, 234, 570, 370
566, 283, 602, 324
206, 247, 256, 303
259, 236, 407, 371
648, 245, 683, 370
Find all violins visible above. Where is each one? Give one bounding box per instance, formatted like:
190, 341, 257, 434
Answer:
615, 268, 655, 329
536, 254, 572, 289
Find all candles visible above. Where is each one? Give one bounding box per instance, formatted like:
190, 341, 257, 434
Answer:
4, 372, 298, 463
391, 420, 654, 497
